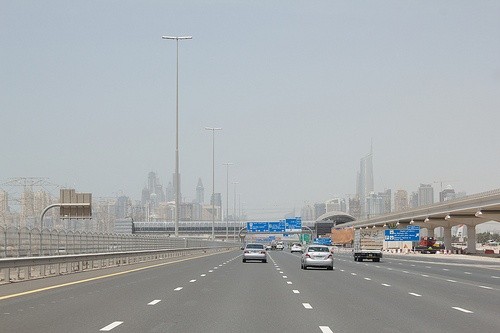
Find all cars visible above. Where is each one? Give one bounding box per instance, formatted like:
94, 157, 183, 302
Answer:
290, 242, 304, 253
264, 240, 284, 251
300, 244, 335, 270
240, 243, 268, 263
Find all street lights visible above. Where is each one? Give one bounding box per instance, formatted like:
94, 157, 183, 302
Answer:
224, 163, 233, 241
162, 35, 193, 237
205, 127, 222, 240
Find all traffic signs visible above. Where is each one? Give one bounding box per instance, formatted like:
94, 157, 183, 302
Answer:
285, 218, 302, 233
268, 219, 285, 233
384, 224, 421, 242
246, 221, 268, 233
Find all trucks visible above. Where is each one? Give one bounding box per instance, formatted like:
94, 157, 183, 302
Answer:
351, 228, 385, 262
